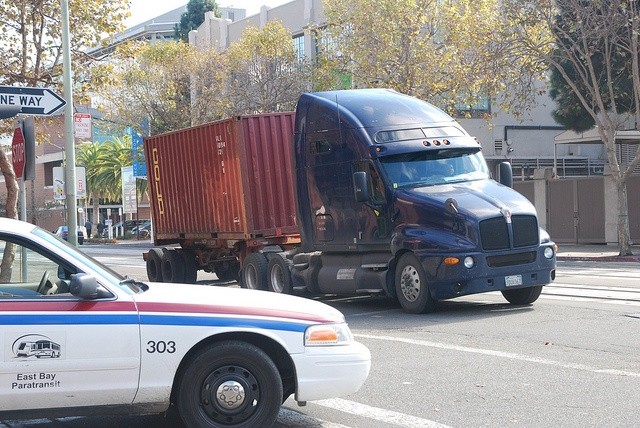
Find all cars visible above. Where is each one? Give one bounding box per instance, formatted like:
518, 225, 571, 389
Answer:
51, 226, 84, 245
102, 220, 149, 239
0, 218, 372, 428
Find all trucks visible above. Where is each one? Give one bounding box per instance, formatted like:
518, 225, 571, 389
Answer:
143, 88, 558, 313
